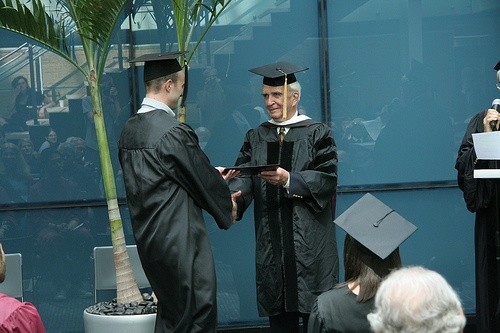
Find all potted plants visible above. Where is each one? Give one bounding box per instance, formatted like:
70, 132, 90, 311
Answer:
0, 0, 157, 333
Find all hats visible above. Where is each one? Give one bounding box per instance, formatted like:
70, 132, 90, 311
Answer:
494, 61, 500, 71
332, 192, 419, 260
248, 61, 309, 118
129, 49, 191, 108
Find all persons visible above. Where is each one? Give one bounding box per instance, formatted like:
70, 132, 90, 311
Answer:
304, 193, 419, 333
119, 49, 244, 333
0, 242, 46, 333
455, 61, 500, 333
368, 267, 465, 333
1, 57, 450, 304
227, 61, 338, 333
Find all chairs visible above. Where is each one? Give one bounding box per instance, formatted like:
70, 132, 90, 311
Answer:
92, 245, 151, 305
0, 253, 24, 303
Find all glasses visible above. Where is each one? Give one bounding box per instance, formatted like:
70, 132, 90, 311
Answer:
495, 82, 500, 90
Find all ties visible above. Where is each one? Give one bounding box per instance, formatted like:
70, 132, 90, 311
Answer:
278, 127, 286, 142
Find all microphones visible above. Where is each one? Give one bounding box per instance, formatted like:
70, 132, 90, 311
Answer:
490, 99, 500, 127
